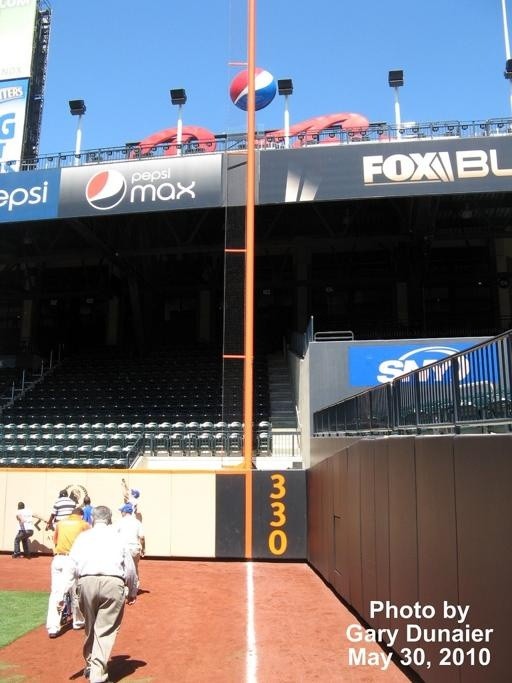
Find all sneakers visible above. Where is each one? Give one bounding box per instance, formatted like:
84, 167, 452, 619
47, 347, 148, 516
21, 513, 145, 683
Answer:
12, 551, 21, 557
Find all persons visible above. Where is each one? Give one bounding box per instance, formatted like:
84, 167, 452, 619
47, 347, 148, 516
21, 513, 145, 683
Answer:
11, 488, 146, 683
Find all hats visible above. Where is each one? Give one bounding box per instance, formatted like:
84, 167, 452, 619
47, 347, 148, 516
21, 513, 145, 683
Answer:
119, 504, 133, 513
131, 489, 139, 498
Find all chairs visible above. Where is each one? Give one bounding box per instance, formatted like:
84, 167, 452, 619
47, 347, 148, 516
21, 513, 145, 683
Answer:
332, 392, 512, 433
0, 340, 270, 469
